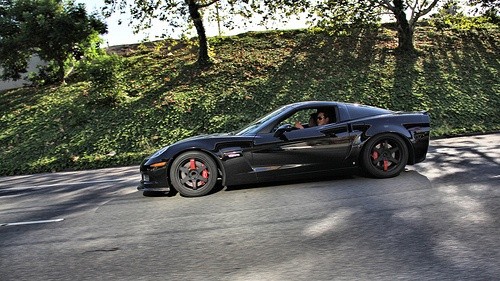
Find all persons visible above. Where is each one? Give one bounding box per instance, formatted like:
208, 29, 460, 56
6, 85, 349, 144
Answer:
294, 111, 331, 129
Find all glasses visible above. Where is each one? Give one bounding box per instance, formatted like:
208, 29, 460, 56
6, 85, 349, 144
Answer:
317, 117, 326, 120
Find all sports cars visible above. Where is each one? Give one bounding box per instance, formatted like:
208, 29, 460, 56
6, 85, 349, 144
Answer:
137, 100, 431, 197
